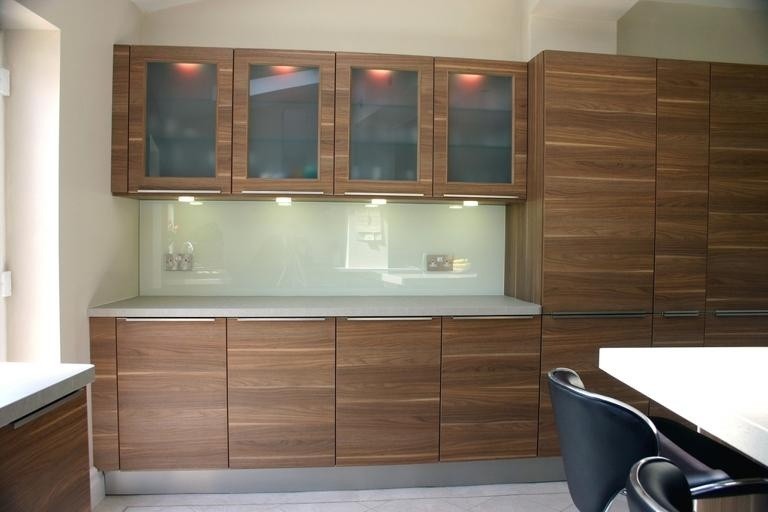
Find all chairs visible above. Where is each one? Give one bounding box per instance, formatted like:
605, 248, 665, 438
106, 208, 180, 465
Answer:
548, 368, 726, 512
630, 454, 768, 512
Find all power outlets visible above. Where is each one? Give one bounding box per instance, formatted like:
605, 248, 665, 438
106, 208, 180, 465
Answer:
164, 253, 191, 271
427, 254, 453, 271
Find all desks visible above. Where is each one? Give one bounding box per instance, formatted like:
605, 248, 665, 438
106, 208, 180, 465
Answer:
595, 344, 768, 472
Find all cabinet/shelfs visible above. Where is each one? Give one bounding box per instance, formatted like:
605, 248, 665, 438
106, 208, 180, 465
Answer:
505, 50, 768, 459
333, 48, 528, 201
110, 45, 333, 200
89, 319, 335, 468
1, 385, 91, 512
336, 321, 540, 466
